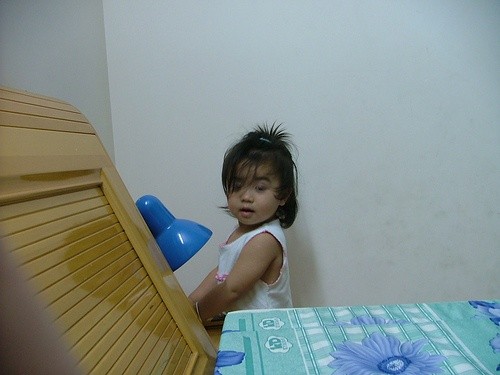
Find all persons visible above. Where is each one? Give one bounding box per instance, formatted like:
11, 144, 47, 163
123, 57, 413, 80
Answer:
182, 120, 299, 325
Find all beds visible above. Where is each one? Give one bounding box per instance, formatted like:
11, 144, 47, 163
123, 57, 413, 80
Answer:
214, 299, 500, 375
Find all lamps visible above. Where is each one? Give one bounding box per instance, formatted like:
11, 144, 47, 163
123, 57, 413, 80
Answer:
134, 194, 213, 274
